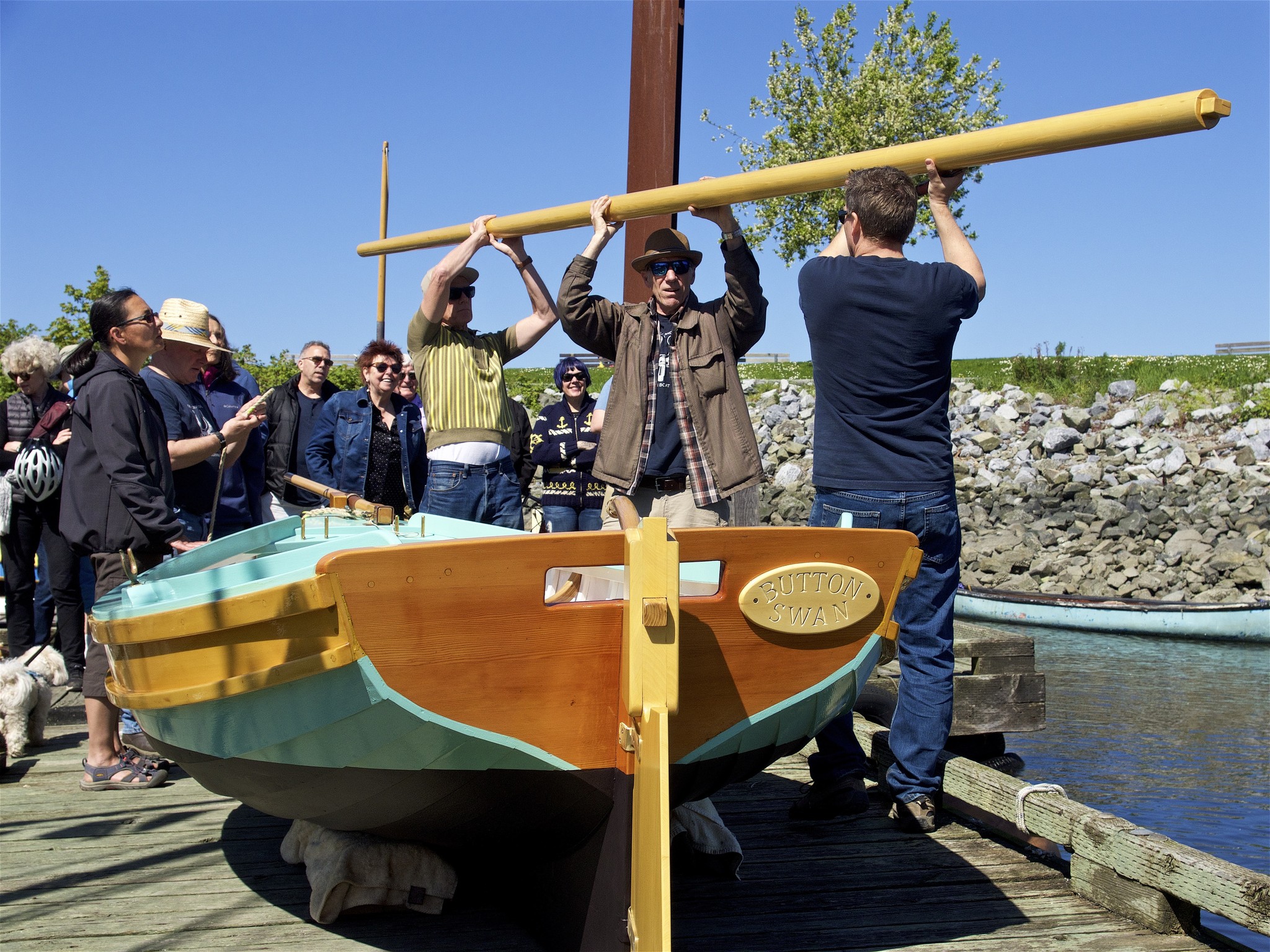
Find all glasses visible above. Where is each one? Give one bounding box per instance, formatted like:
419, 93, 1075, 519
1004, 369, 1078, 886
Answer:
561, 372, 585, 382
302, 356, 333, 366
399, 372, 416, 380
8, 366, 42, 381
837, 208, 849, 224
111, 311, 159, 338
367, 361, 402, 374
647, 259, 691, 276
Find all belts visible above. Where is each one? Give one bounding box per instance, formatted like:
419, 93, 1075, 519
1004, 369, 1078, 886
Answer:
639, 472, 690, 493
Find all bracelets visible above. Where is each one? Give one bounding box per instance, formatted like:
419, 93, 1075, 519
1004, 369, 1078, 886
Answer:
215, 431, 226, 450
722, 226, 744, 240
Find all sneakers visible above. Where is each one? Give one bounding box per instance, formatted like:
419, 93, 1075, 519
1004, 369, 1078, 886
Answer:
808, 752, 866, 797
892, 794, 936, 832
65, 665, 86, 692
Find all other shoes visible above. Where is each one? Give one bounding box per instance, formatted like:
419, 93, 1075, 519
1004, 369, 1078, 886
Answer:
122, 733, 160, 756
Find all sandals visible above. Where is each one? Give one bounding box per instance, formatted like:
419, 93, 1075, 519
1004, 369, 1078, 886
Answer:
119, 746, 169, 772
79, 755, 168, 791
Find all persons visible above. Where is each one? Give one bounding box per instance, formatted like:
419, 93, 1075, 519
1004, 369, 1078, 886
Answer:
305, 339, 429, 520
556, 177, 768, 532
230, 359, 268, 526
407, 215, 560, 529
122, 299, 267, 753
0, 338, 87, 690
34, 345, 98, 659
797, 158, 986, 831
590, 374, 614, 433
508, 397, 538, 491
260, 341, 342, 523
530, 357, 608, 532
392, 354, 426, 433
189, 314, 263, 540
58, 288, 211, 791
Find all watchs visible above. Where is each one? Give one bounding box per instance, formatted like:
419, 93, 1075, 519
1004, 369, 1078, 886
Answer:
515, 255, 533, 269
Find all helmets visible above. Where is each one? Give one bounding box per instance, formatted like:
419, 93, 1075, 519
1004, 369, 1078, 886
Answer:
14, 443, 63, 502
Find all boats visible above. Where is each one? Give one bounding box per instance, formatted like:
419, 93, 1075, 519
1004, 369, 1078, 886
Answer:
85, 489, 927, 863
952, 584, 1270, 646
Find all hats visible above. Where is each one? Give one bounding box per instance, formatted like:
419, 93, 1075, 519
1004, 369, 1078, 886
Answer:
631, 229, 703, 274
157, 298, 234, 353
58, 338, 95, 368
